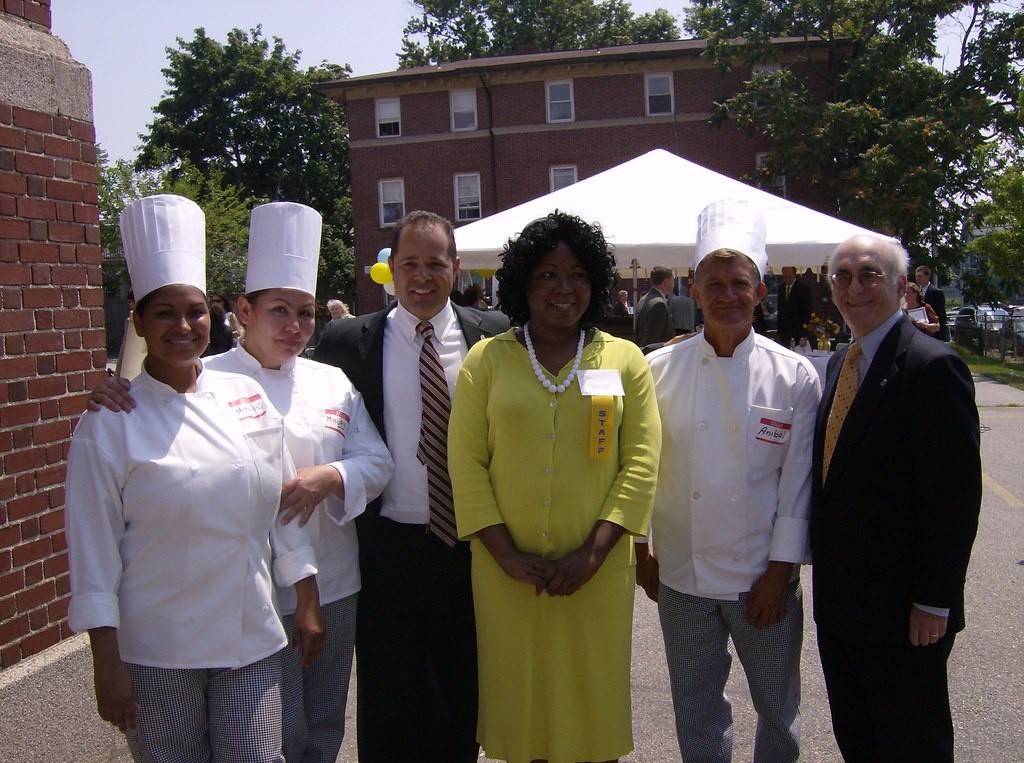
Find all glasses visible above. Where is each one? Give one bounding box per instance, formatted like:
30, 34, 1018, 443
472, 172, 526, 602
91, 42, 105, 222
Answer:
828, 271, 884, 289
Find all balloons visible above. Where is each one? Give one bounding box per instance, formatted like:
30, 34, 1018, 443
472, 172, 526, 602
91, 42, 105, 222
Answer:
370, 243, 395, 295
478, 270, 496, 278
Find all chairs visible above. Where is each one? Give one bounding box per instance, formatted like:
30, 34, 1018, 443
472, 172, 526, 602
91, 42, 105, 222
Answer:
835, 342, 850, 352
791, 340, 813, 358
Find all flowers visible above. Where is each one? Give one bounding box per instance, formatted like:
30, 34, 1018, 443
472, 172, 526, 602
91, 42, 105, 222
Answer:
802, 312, 842, 336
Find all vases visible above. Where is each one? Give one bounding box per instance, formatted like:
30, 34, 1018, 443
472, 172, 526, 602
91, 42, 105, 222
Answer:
817, 337, 833, 353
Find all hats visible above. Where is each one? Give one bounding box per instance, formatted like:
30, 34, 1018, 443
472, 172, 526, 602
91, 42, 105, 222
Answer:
245, 202, 322, 298
120, 195, 206, 305
327, 300, 347, 314
694, 199, 768, 282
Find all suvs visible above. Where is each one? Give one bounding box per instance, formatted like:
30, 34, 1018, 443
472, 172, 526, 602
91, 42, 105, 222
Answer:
953, 305, 1013, 351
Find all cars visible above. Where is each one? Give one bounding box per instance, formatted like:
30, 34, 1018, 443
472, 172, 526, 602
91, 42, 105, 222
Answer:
947, 310, 959, 336
997, 318, 1024, 356
1002, 305, 1024, 319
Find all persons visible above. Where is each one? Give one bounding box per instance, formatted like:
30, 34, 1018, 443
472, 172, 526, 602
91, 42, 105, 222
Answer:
66, 193, 398, 763
812, 235, 982, 763
449, 283, 488, 311
633, 197, 823, 763
315, 211, 515, 763
611, 265, 822, 348
448, 210, 662, 763
901, 265, 951, 341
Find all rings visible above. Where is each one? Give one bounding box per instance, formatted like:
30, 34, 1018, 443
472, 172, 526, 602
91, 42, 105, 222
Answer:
930, 633, 939, 638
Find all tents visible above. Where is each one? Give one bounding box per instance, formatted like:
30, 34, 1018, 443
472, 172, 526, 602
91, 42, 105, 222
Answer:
385, 148, 904, 340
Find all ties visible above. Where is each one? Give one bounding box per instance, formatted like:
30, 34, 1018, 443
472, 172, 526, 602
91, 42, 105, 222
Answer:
823, 340, 862, 486
787, 287, 790, 294
416, 322, 458, 547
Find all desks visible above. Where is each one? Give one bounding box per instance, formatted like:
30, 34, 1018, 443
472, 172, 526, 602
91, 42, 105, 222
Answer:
799, 351, 838, 390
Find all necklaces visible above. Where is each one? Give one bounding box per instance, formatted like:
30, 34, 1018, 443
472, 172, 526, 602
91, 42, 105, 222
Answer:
523, 321, 585, 393
